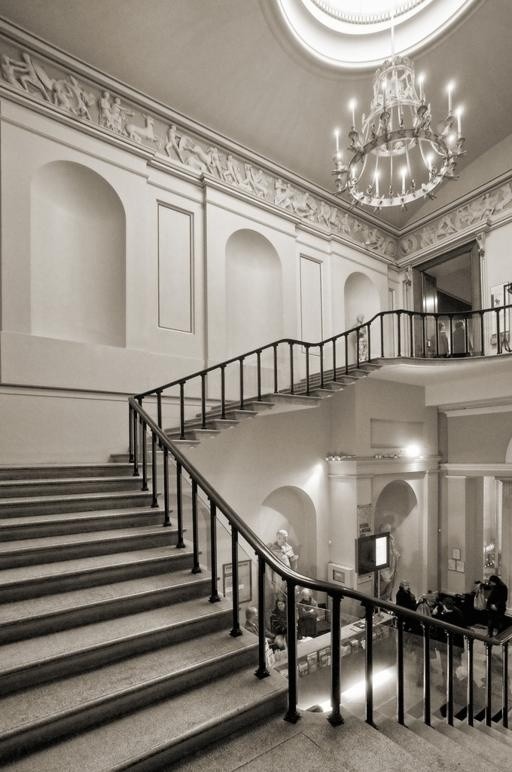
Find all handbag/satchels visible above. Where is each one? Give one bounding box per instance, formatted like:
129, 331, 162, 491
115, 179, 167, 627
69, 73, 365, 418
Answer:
474, 593, 487, 611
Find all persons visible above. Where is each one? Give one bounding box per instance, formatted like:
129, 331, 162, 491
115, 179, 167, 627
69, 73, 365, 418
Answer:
297, 588, 318, 641
353, 315, 368, 362
270, 599, 288, 650
377, 524, 397, 600
264, 530, 298, 605
243, 607, 275, 646
430, 320, 449, 358
452, 320, 473, 358
396, 574, 508, 696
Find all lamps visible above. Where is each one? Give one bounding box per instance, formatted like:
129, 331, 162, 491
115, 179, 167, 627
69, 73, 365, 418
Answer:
330, 4, 466, 212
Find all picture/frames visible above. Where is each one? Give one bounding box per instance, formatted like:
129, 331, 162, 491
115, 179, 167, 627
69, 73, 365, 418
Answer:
223, 559, 252, 604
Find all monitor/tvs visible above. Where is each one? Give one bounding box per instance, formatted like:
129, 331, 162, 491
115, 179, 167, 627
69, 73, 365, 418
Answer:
355, 532, 390, 576
